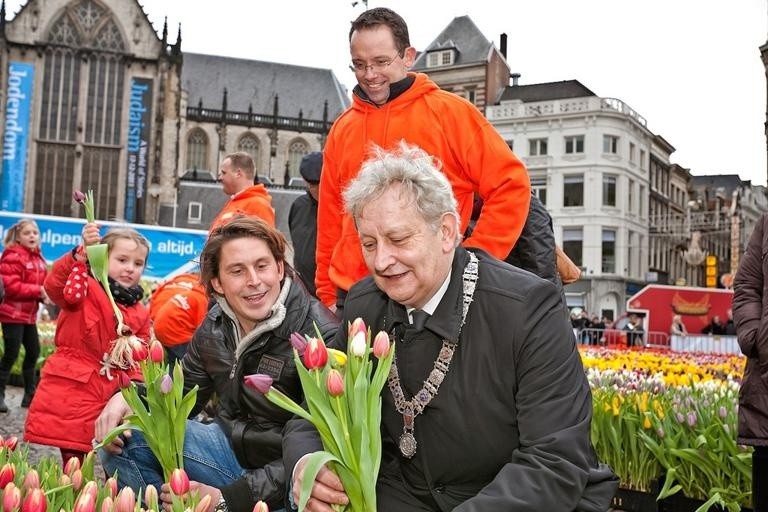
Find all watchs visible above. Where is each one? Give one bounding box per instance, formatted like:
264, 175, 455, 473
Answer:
214, 490, 229, 511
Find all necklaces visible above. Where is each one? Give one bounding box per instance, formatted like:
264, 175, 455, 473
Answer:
379, 249, 480, 458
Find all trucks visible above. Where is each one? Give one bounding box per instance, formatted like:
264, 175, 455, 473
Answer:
599, 281, 734, 354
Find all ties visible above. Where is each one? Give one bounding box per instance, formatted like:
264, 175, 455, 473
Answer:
410, 310, 429, 332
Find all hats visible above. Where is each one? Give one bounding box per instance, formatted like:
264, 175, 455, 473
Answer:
300, 151, 323, 185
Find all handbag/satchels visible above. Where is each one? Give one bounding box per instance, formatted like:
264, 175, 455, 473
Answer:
555, 245, 581, 285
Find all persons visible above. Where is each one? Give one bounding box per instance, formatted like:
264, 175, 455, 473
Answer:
152, 284, 211, 378
91, 215, 345, 511
733, 203, 768, 512
317, 6, 531, 316
560, 298, 739, 352
23, 223, 152, 473
146, 268, 205, 370
204, 151, 275, 245
0, 219, 48, 414
287, 151, 328, 299
280, 143, 620, 512
459, 184, 565, 313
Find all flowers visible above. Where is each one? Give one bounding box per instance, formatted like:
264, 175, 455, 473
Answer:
92, 337, 200, 484
70, 186, 149, 371
245, 315, 396, 512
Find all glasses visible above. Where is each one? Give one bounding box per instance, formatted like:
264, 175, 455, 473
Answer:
349, 49, 403, 73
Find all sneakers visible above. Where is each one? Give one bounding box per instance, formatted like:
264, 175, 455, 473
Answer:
21, 393, 35, 408
0, 396, 8, 413
